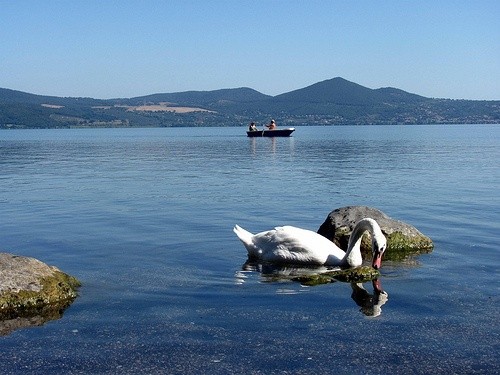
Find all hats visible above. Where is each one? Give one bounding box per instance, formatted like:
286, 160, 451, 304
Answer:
251, 121, 256, 123
271, 120, 275, 123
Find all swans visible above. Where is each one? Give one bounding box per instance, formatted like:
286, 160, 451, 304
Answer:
231, 217, 389, 277
233, 260, 388, 318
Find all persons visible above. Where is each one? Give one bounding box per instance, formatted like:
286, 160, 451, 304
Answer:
265, 119, 276, 130
249, 121, 259, 131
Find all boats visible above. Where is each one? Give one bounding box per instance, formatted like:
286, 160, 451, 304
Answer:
245, 127, 295, 137
